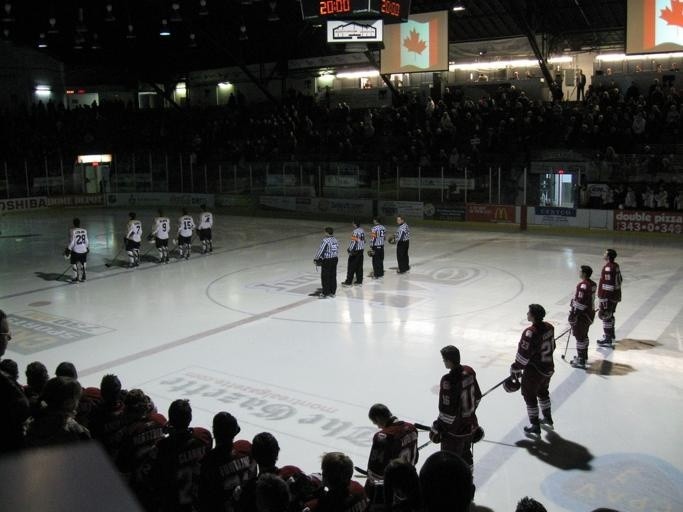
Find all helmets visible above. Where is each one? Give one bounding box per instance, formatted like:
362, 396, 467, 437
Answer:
598, 308, 613, 320
504, 377, 520, 392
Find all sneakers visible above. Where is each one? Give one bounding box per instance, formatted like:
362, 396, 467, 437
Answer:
524, 426, 541, 434
570, 361, 586, 368
603, 333, 616, 339
597, 339, 613, 347
538, 417, 553, 426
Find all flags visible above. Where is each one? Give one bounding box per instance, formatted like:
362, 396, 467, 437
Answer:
643, 0, 683, 50
391, 16, 438, 69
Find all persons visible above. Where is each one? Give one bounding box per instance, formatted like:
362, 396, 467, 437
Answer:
1, 304, 556, 511
313, 227, 339, 298
2, 65, 682, 212
568, 265, 597, 369
195, 204, 214, 255
151, 209, 171, 263
63, 218, 90, 284
341, 220, 366, 286
368, 217, 386, 278
176, 209, 196, 259
124, 212, 143, 268
388, 215, 411, 274
597, 249, 623, 344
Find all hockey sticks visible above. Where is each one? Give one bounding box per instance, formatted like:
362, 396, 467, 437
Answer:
55, 264, 72, 280
414, 375, 511, 430
561, 328, 570, 359
354, 440, 433, 475
105, 243, 125, 267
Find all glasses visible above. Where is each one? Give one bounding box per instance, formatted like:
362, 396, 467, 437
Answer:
0, 331, 11, 340
